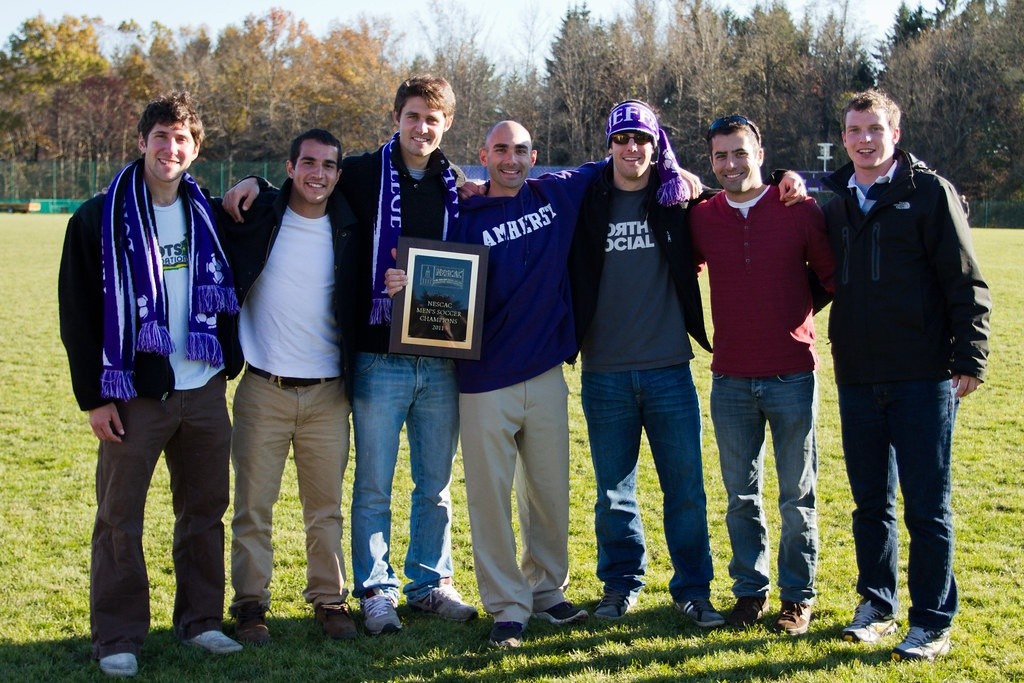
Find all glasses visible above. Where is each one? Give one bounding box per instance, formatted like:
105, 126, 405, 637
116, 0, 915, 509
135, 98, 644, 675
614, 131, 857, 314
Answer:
611, 133, 652, 145
708, 116, 759, 143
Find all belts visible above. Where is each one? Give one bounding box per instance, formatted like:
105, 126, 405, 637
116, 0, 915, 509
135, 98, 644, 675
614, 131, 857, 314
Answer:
246, 361, 339, 387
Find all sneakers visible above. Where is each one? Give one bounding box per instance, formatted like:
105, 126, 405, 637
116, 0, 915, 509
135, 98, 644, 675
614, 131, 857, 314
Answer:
489, 622, 522, 647
360, 588, 403, 634
316, 603, 356, 640
842, 600, 899, 642
532, 601, 589, 625
91, 653, 138, 676
237, 611, 271, 643
725, 594, 768, 625
172, 630, 243, 653
675, 598, 725, 626
418, 577, 479, 622
891, 627, 950, 661
776, 601, 811, 634
595, 591, 639, 619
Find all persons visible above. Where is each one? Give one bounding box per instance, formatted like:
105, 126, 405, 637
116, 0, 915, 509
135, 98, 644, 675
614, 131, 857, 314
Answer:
57, 94, 244, 675
385, 118, 704, 645
224, 73, 479, 635
459, 100, 808, 627
691, 115, 832, 632
93, 128, 359, 638
807, 90, 989, 661
